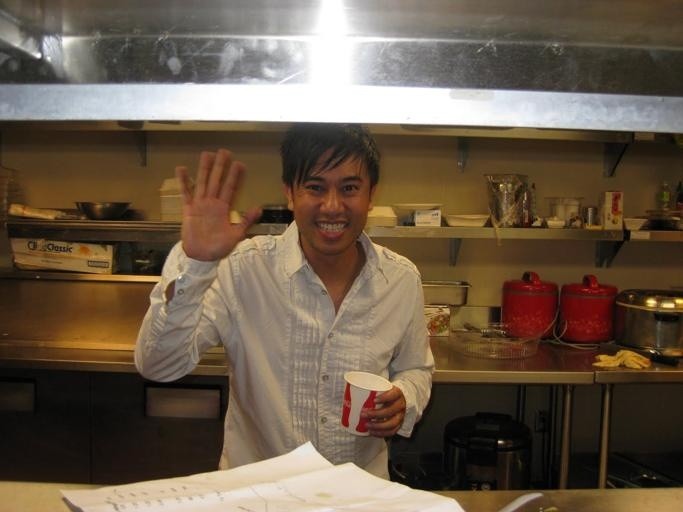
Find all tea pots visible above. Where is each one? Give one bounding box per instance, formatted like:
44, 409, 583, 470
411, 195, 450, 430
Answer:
482, 171, 529, 229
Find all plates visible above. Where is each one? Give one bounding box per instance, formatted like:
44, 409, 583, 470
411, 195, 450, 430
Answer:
585, 224, 603, 231
393, 201, 445, 211
442, 213, 492, 227
362, 205, 398, 229
159, 176, 199, 225
544, 218, 567, 228
530, 217, 543, 226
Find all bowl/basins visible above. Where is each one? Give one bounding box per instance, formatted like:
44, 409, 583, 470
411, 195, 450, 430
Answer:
72, 199, 133, 221
623, 218, 648, 231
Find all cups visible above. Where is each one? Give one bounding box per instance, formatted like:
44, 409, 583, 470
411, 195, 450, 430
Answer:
339, 370, 395, 438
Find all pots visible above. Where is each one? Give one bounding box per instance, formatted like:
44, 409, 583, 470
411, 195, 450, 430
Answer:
615, 287, 683, 359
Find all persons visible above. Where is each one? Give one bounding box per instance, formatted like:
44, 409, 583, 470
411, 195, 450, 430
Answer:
129, 123, 437, 483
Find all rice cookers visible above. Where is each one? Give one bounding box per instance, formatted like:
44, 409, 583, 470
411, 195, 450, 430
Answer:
441, 410, 536, 493
560, 270, 620, 343
498, 270, 561, 344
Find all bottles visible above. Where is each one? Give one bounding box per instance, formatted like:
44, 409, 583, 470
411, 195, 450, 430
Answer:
581, 206, 598, 226
659, 181, 671, 210
675, 182, 683, 209
0, 166, 18, 272
